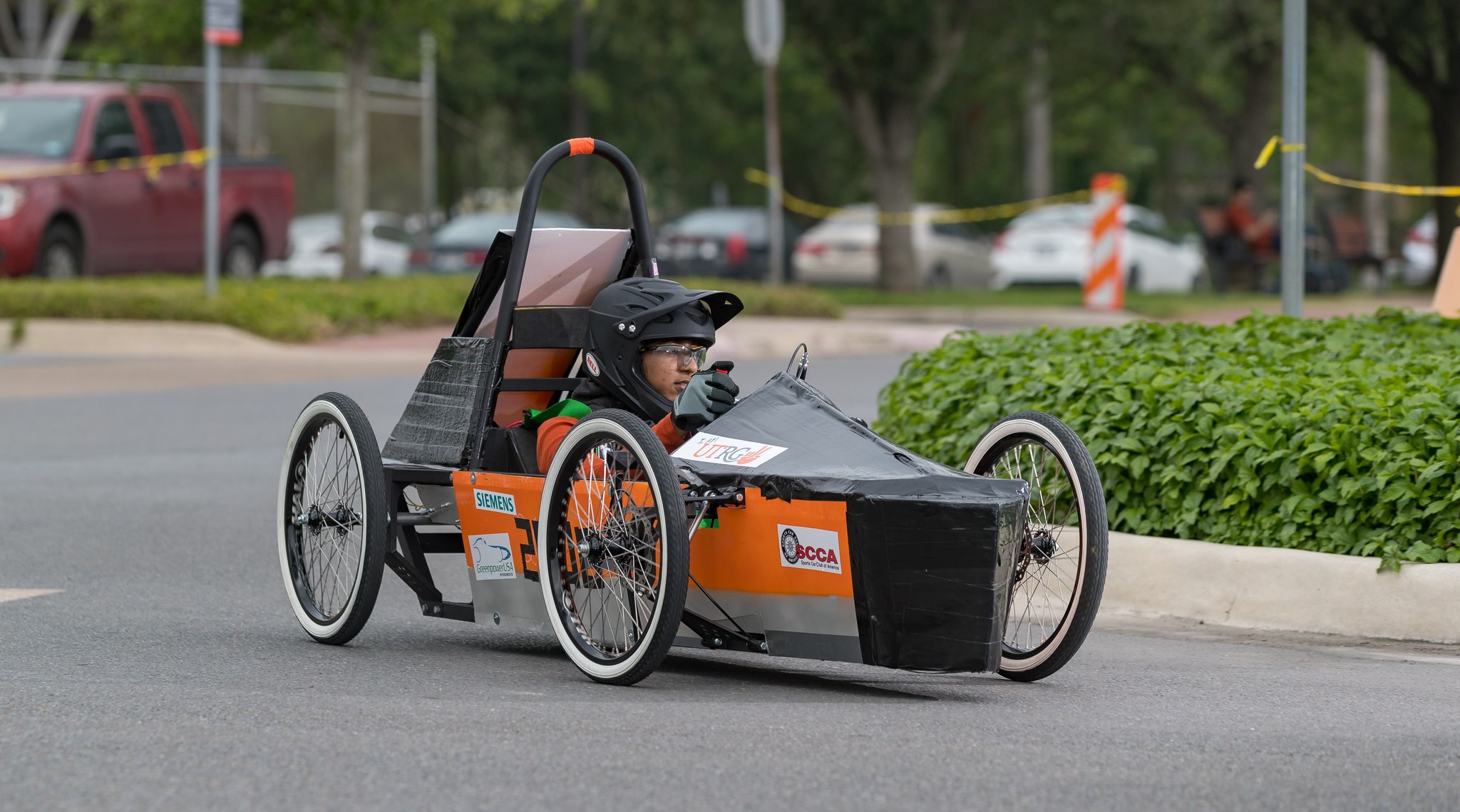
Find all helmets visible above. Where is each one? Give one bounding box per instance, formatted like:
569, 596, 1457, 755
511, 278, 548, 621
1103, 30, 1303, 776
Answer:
582, 277, 744, 426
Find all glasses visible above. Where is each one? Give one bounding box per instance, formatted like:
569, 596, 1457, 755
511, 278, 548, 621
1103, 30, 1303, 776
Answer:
639, 344, 706, 370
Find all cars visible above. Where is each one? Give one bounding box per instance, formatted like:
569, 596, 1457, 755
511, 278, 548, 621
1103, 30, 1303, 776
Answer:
793, 197, 1000, 292
408, 206, 594, 276
990, 198, 1210, 298
646, 203, 803, 279
259, 209, 413, 282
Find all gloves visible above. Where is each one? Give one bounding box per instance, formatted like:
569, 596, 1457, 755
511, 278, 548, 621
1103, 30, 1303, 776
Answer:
671, 361, 740, 431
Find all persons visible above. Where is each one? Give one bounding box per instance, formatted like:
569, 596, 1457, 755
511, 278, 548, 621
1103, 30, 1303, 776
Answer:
539, 277, 743, 475
1221, 176, 1324, 293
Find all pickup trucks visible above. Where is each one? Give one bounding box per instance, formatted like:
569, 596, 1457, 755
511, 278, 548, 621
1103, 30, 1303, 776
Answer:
2, 78, 319, 281
